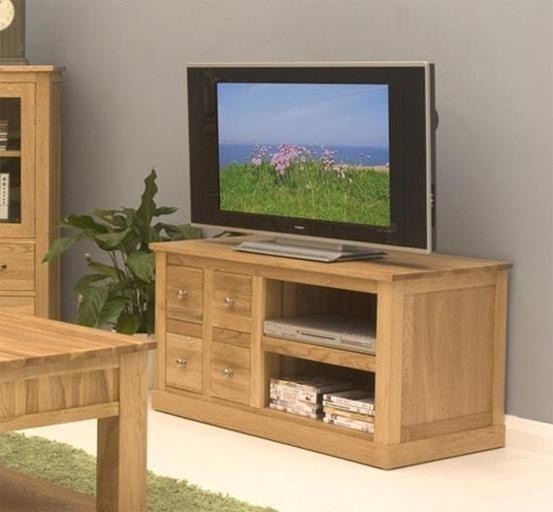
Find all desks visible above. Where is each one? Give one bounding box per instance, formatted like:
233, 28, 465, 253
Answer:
0, 308, 157, 512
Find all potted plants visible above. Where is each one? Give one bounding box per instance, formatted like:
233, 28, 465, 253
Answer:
41, 167, 246, 390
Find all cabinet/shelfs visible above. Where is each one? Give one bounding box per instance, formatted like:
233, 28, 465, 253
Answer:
0, 66, 67, 320
147, 239, 512, 470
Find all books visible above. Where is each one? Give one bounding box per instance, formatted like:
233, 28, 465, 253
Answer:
268, 377, 373, 435
1, 118, 21, 223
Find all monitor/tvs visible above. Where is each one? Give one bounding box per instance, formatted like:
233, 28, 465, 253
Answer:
183, 60, 439, 264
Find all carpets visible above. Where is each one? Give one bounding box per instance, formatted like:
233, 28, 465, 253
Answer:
0, 432, 278, 512
